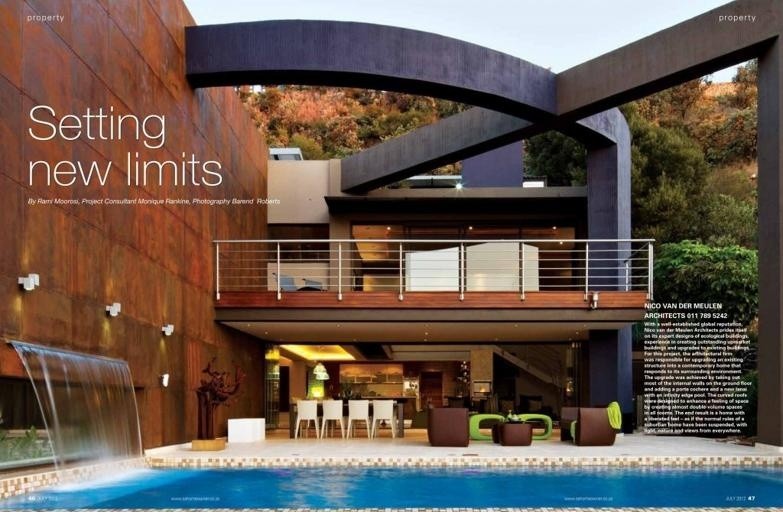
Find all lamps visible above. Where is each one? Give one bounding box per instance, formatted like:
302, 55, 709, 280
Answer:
105, 302, 122, 317
161, 325, 175, 337
313, 360, 330, 380
17, 273, 39, 291
590, 291, 600, 310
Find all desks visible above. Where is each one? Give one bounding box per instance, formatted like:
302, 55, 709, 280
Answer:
289, 403, 405, 438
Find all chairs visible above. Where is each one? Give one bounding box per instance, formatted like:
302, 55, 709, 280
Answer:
272, 272, 327, 291
293, 399, 398, 439
560, 401, 618, 447
427, 404, 470, 447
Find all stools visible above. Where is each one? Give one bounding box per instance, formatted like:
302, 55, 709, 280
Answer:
469, 414, 553, 440
